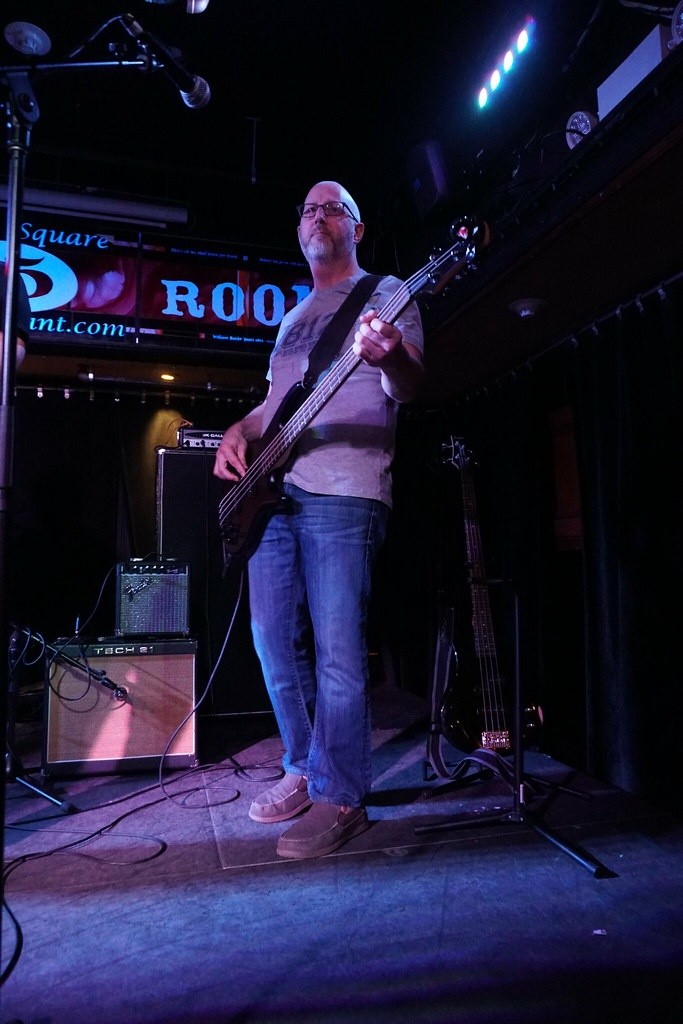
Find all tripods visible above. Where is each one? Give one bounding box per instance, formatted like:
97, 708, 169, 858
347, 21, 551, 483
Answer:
412, 575, 605, 877
0, 618, 120, 815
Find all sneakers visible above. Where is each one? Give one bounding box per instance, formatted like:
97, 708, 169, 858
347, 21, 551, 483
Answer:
276, 800, 368, 859
248, 771, 314, 823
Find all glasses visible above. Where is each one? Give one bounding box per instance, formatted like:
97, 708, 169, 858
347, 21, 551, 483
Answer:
296, 201, 358, 223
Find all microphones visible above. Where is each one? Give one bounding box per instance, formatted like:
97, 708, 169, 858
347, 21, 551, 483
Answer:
120, 13, 210, 108
145, 0, 209, 13
114, 687, 128, 702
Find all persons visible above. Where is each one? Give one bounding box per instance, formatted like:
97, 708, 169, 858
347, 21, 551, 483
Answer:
212, 181, 423, 858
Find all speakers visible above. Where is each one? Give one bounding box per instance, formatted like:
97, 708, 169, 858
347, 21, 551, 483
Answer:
37, 448, 275, 777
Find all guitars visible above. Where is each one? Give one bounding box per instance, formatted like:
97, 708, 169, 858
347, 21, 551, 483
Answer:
218, 210, 491, 564
435, 429, 547, 760
